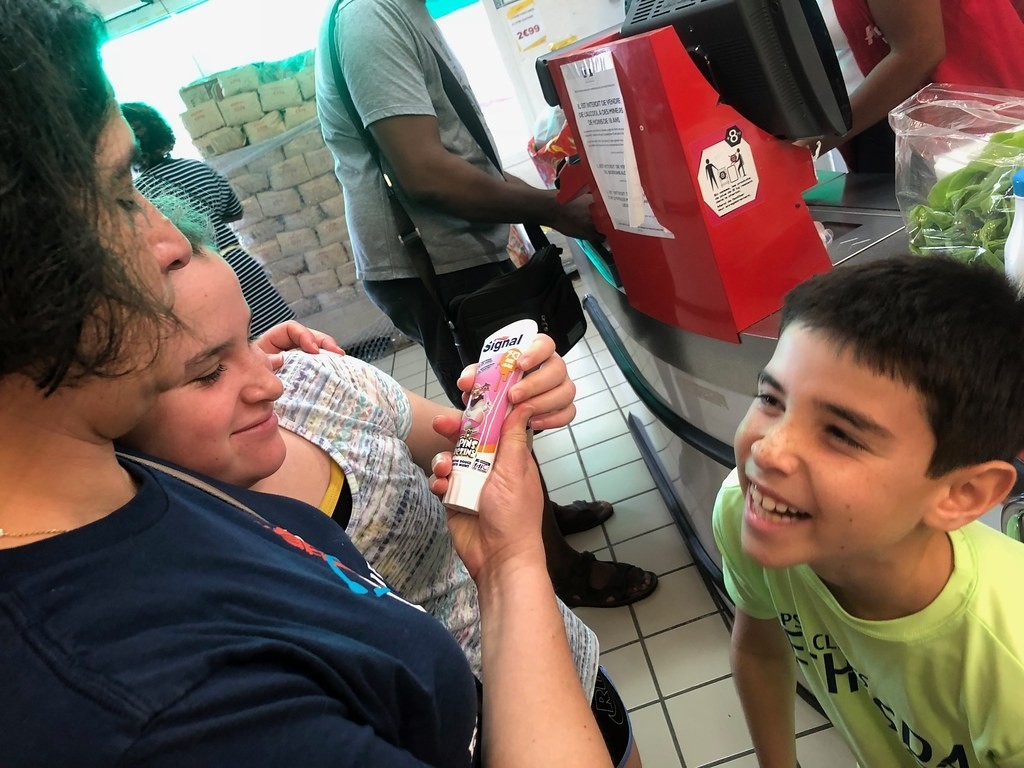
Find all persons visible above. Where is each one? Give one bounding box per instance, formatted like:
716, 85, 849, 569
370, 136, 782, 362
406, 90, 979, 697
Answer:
709, 251, 1024, 768
790, 1, 1024, 176
115, 101, 298, 346
313, 0, 663, 609
0, 0, 618, 768
110, 196, 651, 768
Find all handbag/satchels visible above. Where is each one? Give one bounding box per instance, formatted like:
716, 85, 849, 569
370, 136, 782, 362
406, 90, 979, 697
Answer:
444, 242, 588, 379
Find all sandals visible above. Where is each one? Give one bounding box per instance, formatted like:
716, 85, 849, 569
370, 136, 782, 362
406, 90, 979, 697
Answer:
557, 500, 614, 535
554, 550, 658, 608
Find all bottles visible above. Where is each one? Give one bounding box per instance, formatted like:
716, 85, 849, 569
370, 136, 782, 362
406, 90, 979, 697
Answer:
1004, 168, 1024, 299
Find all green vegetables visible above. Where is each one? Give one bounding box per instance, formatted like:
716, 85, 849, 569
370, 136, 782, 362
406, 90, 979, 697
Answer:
905, 128, 1024, 275
